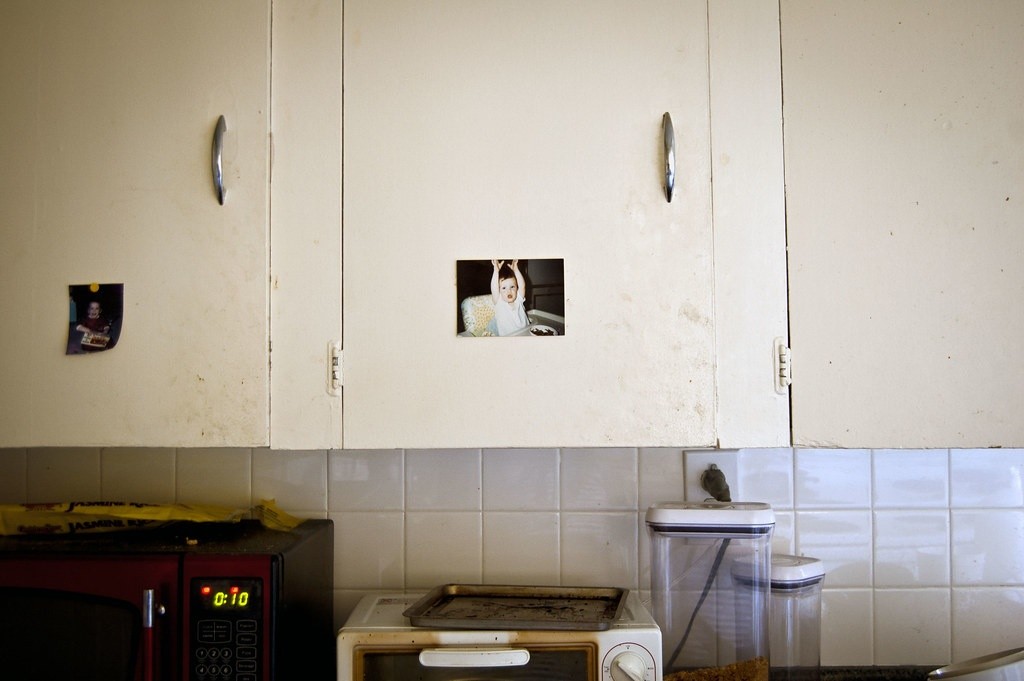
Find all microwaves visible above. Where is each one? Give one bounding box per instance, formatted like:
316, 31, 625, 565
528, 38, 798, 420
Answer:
337, 591, 662, 681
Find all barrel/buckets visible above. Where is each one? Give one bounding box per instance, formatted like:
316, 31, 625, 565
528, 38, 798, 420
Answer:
737, 552, 822, 681
645, 501, 775, 681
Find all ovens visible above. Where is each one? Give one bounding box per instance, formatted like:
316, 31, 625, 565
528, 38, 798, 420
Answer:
0, 503, 337, 681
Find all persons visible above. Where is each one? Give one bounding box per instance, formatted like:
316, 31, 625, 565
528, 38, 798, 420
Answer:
490, 259, 532, 337
75, 298, 114, 352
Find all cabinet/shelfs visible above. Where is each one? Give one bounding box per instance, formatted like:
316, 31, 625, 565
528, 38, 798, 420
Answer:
1, 0, 270, 450
340, 3, 718, 450
782, 2, 1024, 451
